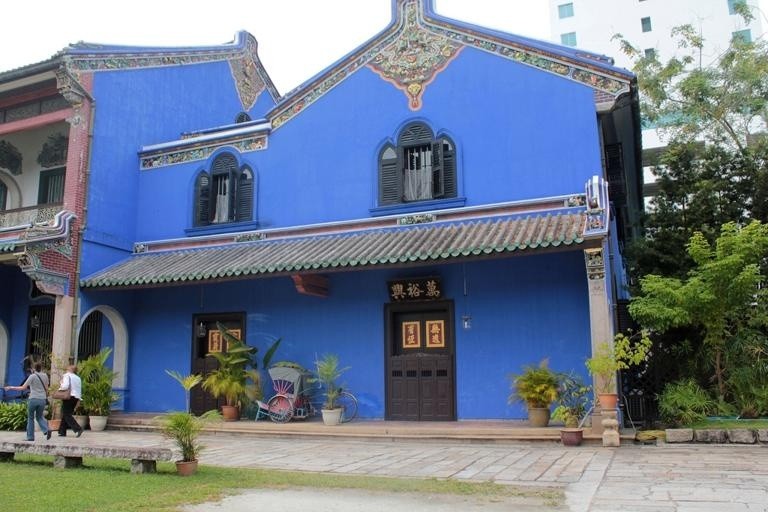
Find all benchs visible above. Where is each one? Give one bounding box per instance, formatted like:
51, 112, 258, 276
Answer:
0, 438, 173, 476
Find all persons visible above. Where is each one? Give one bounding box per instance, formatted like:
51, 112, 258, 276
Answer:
57, 365, 83, 437
4, 362, 52, 441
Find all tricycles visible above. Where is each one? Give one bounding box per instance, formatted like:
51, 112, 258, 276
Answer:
252, 361, 358, 423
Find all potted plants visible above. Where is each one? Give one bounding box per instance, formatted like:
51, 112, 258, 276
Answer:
47, 345, 122, 432
307, 352, 351, 425
199, 319, 262, 422
152, 411, 224, 475
505, 326, 655, 447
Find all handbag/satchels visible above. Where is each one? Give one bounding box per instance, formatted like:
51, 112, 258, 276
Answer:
44, 385, 49, 396
53, 376, 71, 400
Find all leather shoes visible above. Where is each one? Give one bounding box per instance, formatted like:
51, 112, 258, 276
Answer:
76, 429, 83, 437
24, 438, 33, 441
47, 430, 50, 439
58, 433, 66, 437
